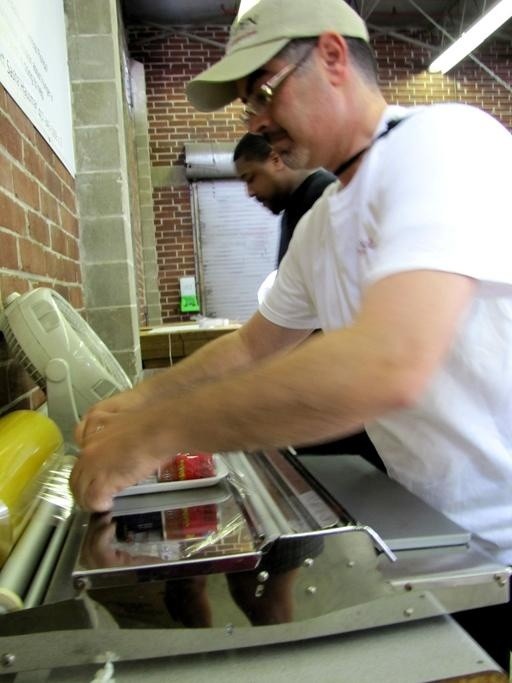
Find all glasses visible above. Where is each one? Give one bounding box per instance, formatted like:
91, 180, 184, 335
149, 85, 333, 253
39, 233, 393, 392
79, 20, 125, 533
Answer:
238, 41, 319, 131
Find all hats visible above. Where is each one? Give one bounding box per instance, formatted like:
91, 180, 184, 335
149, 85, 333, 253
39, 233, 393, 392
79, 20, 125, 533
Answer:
186, 0, 370, 111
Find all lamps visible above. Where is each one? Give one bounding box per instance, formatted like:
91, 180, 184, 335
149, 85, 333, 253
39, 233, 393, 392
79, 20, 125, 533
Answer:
428, 1, 512, 78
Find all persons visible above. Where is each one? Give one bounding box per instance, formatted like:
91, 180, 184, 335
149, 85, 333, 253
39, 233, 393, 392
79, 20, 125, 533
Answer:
234, 133, 386, 474
68, 0, 512, 676
